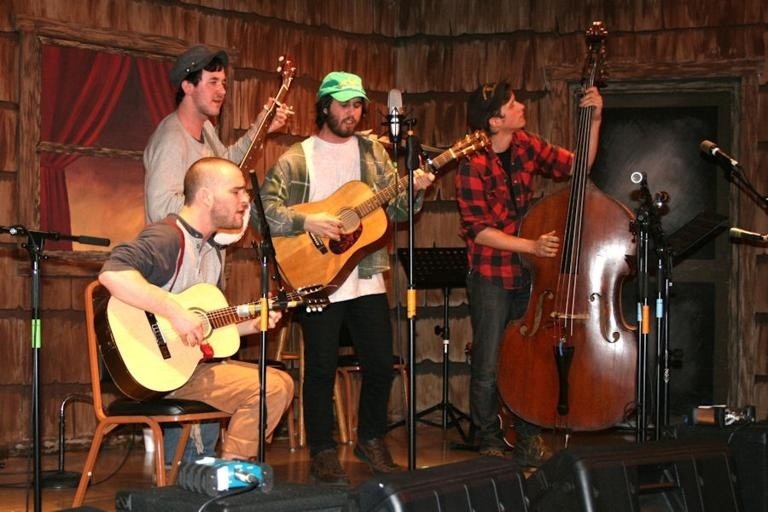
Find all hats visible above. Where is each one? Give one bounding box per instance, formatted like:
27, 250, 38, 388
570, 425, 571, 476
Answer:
318, 71, 372, 104
167, 44, 230, 89
465, 77, 510, 130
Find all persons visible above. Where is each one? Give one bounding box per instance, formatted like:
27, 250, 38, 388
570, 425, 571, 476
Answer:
455, 80, 603, 458
250, 70, 435, 487
98, 156, 295, 461
142, 45, 295, 472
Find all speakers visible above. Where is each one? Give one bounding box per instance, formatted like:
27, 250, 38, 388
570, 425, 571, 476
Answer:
727, 420, 766, 509
531, 438, 741, 511
356, 450, 536, 511
112, 482, 350, 510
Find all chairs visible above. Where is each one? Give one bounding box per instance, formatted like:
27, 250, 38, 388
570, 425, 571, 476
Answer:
236, 292, 409, 453
72, 279, 232, 508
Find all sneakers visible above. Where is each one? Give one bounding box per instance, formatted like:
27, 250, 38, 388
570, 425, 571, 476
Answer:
511, 429, 555, 467
354, 438, 401, 476
307, 449, 352, 483
466, 425, 516, 456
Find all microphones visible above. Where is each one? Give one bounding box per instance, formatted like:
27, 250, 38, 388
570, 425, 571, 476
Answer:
272, 275, 295, 320
697, 138, 739, 167
388, 88, 403, 144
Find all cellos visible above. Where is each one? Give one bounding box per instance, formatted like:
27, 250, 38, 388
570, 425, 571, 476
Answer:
495, 20, 641, 434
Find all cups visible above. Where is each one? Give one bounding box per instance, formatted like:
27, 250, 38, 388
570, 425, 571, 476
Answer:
743, 405, 756, 423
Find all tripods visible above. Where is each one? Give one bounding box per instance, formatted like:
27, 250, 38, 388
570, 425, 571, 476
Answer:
388, 290, 471, 443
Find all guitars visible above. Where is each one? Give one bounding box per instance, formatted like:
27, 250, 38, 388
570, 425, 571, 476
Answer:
93, 282, 339, 401
270, 129, 490, 294
